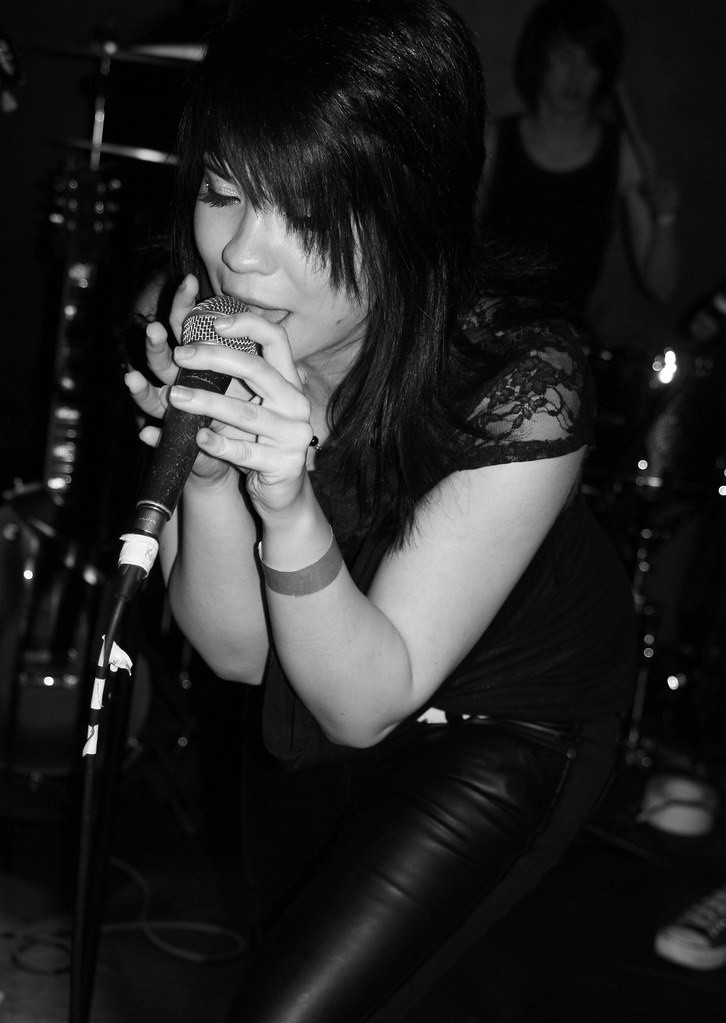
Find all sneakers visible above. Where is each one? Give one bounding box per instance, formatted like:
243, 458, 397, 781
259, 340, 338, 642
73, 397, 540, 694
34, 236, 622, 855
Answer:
639, 724, 719, 834
653, 878, 726, 972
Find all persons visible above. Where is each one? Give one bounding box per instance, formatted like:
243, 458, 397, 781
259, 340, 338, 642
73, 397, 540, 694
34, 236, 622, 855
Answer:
120, 0, 636, 1023
469, 0, 679, 324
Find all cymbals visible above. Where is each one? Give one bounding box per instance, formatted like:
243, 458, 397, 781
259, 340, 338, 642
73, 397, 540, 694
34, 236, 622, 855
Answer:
15, 29, 174, 69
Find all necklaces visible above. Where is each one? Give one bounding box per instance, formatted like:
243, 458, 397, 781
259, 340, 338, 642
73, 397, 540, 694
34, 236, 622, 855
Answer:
309, 436, 322, 451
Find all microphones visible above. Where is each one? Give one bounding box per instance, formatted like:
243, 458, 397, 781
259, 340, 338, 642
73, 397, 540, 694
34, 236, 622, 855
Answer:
115, 295, 262, 598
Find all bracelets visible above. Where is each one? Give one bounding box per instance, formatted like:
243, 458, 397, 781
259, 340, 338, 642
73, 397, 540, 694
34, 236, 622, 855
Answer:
254, 525, 345, 598
